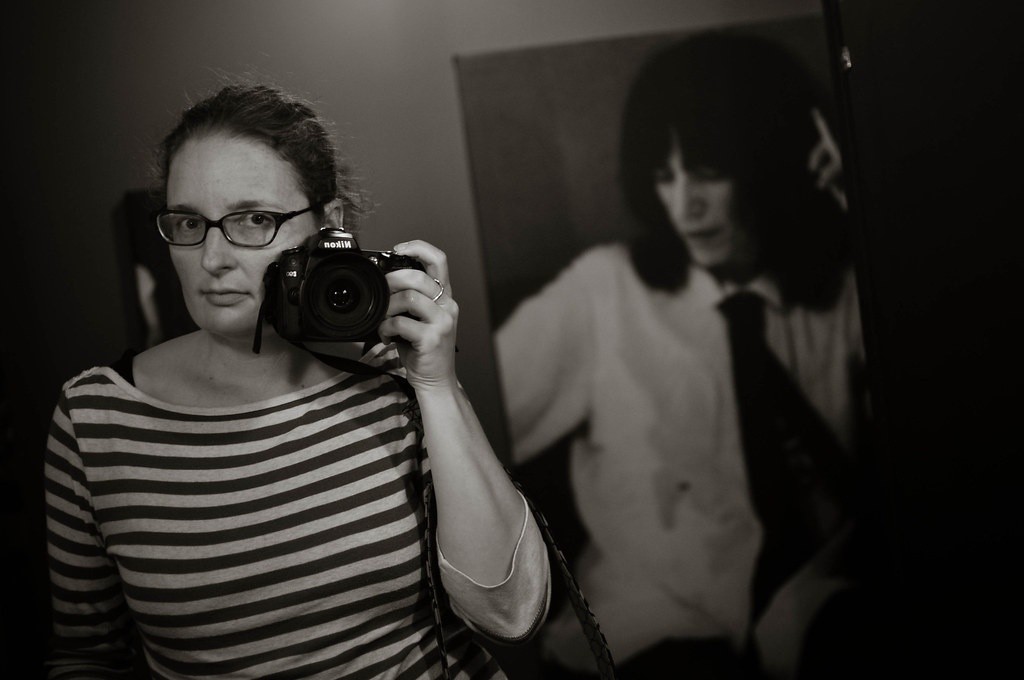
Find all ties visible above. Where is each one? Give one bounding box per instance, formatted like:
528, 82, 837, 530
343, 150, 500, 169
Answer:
718, 290, 860, 646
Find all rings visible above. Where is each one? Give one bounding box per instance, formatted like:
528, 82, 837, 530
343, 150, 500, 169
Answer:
433, 279, 445, 302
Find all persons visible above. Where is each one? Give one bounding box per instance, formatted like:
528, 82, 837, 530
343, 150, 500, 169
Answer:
492, 29, 865, 680
45, 85, 557, 680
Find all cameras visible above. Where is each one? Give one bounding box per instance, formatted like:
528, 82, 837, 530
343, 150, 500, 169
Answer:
262, 225, 428, 345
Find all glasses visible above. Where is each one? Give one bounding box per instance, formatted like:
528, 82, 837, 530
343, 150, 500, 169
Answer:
150, 200, 326, 248
